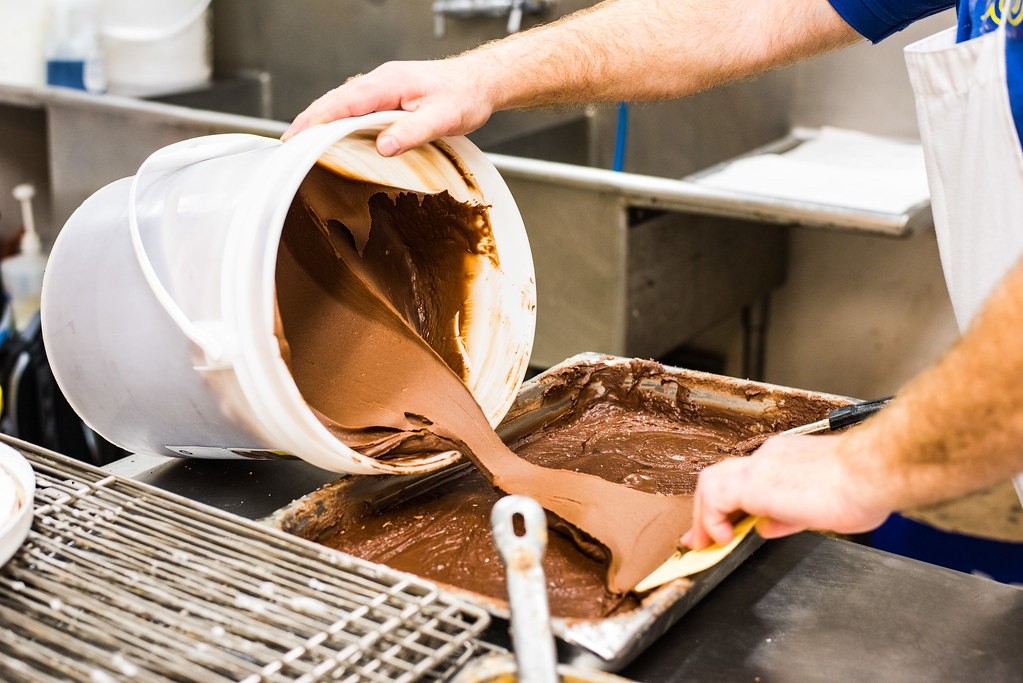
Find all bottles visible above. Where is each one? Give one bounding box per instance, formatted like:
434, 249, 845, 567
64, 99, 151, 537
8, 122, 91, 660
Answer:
1, 235, 49, 340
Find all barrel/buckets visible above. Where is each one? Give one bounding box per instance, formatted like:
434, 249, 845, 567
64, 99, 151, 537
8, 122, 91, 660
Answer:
40, 110, 540, 474
95, 0, 215, 97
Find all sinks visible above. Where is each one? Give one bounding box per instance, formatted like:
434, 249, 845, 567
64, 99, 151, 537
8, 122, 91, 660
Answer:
473, 110, 791, 371
43, 61, 344, 242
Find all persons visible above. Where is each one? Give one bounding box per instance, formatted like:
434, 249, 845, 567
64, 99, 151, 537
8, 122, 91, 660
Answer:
280, 0, 1023, 550
